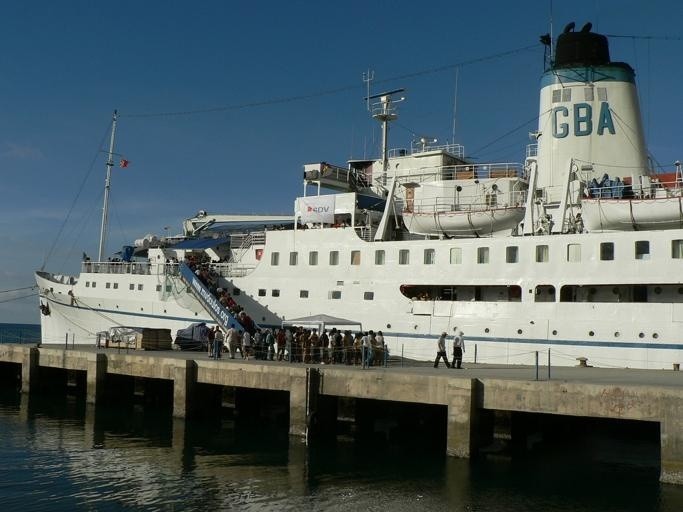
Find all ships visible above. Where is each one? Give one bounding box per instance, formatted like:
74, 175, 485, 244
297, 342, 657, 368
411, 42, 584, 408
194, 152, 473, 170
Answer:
32, 19, 683, 372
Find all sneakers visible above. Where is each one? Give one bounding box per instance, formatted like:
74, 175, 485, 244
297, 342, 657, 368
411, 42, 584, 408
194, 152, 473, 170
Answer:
208, 355, 384, 366
446, 364, 463, 369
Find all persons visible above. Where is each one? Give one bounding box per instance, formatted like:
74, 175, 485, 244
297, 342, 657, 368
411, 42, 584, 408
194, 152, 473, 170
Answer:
264, 219, 349, 231
105, 257, 124, 273
590, 173, 624, 199
575, 213, 584, 234
433, 332, 451, 369
356, 220, 365, 226
165, 255, 385, 369
451, 332, 466, 369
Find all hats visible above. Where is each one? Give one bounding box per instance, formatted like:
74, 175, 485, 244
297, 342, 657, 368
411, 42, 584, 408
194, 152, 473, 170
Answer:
459, 331, 464, 335
442, 331, 448, 335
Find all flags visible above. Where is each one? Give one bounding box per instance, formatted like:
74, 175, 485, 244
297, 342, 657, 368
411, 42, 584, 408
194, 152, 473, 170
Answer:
301, 197, 335, 224
